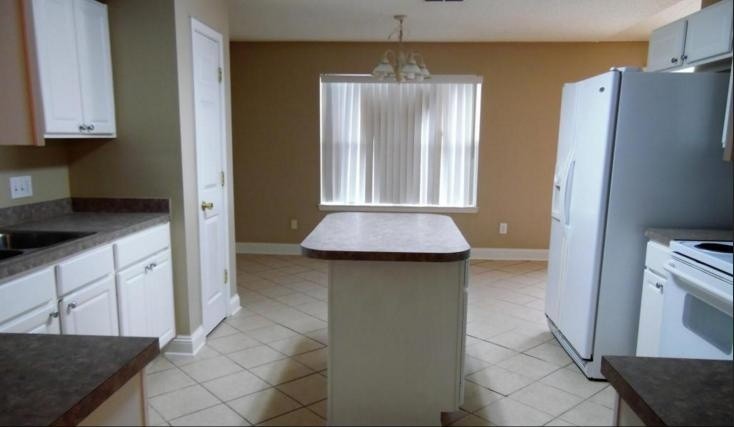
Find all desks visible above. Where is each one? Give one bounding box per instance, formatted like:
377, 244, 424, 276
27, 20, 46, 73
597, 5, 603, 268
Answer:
0, 334, 159, 427
300, 213, 471, 427
601, 355, 734, 427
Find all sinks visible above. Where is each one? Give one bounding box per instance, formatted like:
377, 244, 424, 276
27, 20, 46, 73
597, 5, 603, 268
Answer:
0, 247, 25, 264
0, 227, 100, 252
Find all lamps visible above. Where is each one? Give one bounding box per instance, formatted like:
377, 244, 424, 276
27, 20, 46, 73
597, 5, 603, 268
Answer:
373, 15, 431, 83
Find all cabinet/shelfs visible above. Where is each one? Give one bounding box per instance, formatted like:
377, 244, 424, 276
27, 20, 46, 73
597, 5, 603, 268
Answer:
24, 0, 117, 139
0, 222, 176, 349
647, 0, 733, 72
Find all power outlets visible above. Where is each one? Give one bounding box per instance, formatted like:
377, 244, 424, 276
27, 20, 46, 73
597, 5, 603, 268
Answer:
291, 220, 298, 229
10, 176, 33, 199
500, 223, 507, 234
